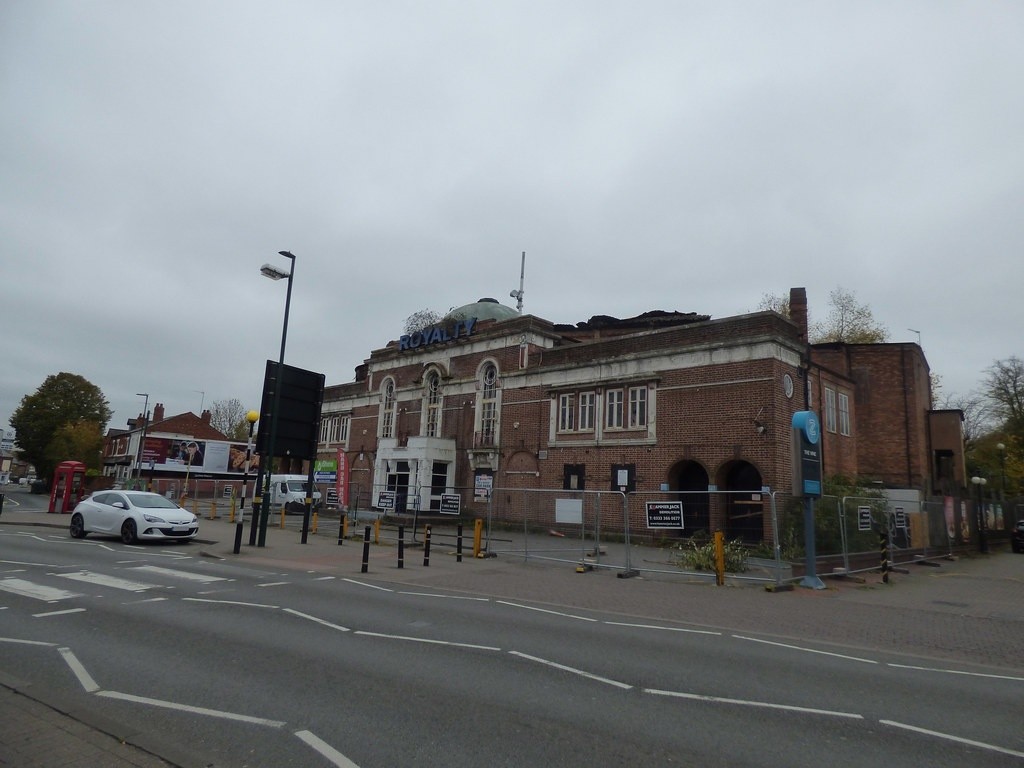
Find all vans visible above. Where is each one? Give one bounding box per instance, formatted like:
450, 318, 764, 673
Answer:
252, 473, 323, 515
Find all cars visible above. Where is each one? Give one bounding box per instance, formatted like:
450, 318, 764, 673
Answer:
1011, 520, 1024, 551
68, 489, 199, 544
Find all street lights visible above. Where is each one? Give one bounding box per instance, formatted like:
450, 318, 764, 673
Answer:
997, 443, 1007, 499
136, 392, 149, 478
971, 476, 990, 553
233, 410, 260, 554
257, 248, 297, 550
